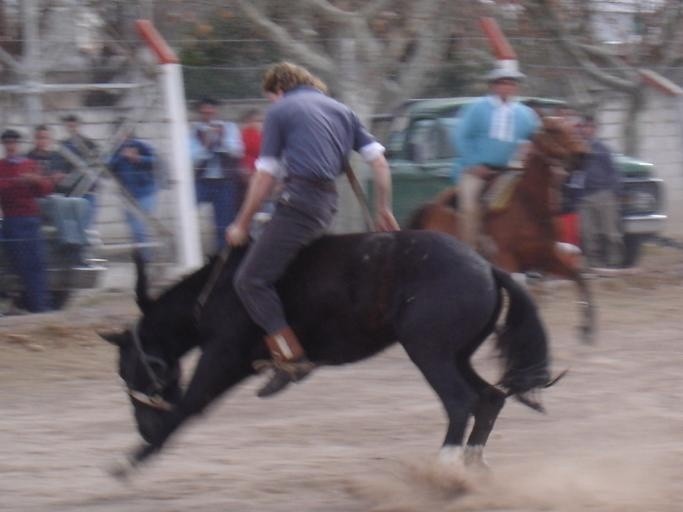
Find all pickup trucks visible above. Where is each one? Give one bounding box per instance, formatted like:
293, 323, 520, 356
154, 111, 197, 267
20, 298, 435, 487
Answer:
353, 96, 667, 270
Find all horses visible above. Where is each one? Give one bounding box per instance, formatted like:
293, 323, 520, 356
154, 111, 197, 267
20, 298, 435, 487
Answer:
404, 102, 598, 347
91, 225, 570, 484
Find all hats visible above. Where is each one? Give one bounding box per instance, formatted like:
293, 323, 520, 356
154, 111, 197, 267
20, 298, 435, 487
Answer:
480, 57, 527, 82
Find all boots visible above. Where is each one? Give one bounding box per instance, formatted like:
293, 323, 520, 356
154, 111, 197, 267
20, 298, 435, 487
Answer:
256, 329, 311, 399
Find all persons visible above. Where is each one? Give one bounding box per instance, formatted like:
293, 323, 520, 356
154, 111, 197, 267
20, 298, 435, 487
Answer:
98, 114, 161, 267
187, 94, 249, 261
223, 60, 398, 398
238, 101, 261, 179
448, 55, 546, 251
573, 117, 639, 271
26, 124, 91, 265
53, 113, 103, 249
0, 129, 67, 316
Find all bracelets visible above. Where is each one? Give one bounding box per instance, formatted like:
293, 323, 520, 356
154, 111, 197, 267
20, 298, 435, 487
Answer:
228, 223, 245, 242
